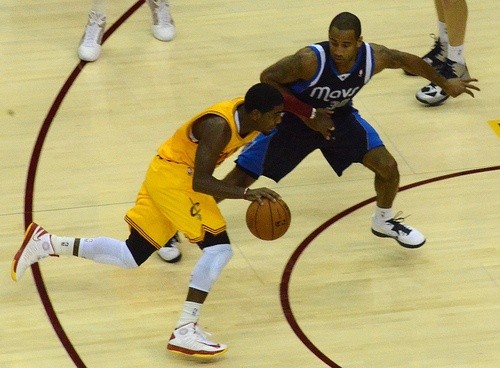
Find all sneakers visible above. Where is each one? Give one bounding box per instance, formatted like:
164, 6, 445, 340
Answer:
77, 9, 106, 62
147, 0, 175, 41
11, 222, 55, 280
372, 210, 425, 248
415, 57, 470, 105
167, 320, 227, 357
404, 34, 446, 76
157, 234, 182, 262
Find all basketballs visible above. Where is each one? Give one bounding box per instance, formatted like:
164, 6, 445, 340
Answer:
245, 196, 292, 240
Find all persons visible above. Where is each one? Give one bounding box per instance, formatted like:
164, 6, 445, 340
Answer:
77, 0, 176, 61
158, 12, 480, 263
404, 0, 471, 104
10, 82, 335, 358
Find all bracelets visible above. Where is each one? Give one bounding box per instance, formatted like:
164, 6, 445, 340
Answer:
242, 185, 249, 199
310, 107, 316, 118
